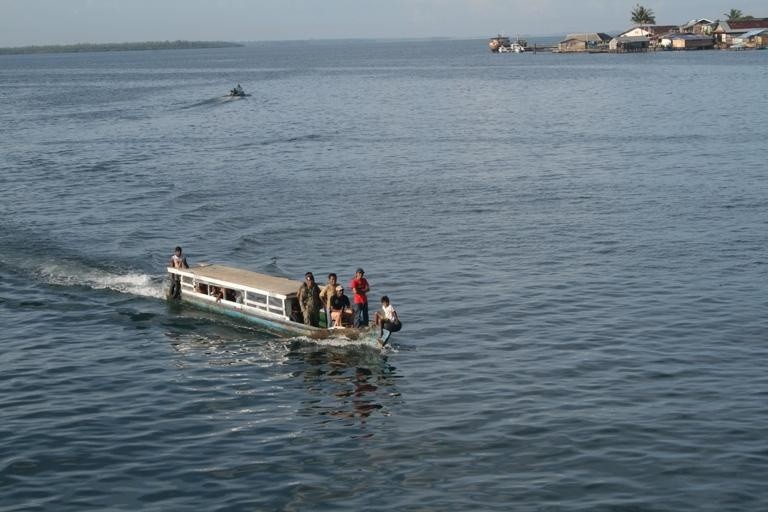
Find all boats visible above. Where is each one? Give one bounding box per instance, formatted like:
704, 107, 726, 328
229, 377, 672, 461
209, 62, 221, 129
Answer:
489, 34, 525, 54
164, 263, 391, 352
222, 83, 251, 100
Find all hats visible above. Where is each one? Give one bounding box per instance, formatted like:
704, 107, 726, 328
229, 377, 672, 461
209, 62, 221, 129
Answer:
337, 286, 344, 292
356, 268, 365, 274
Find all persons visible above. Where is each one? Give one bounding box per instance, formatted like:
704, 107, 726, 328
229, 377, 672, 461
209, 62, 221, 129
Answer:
298, 274, 323, 327
200, 283, 236, 304
350, 269, 370, 328
375, 295, 398, 338
170, 247, 189, 287
297, 272, 312, 323
330, 285, 351, 327
319, 273, 340, 328
